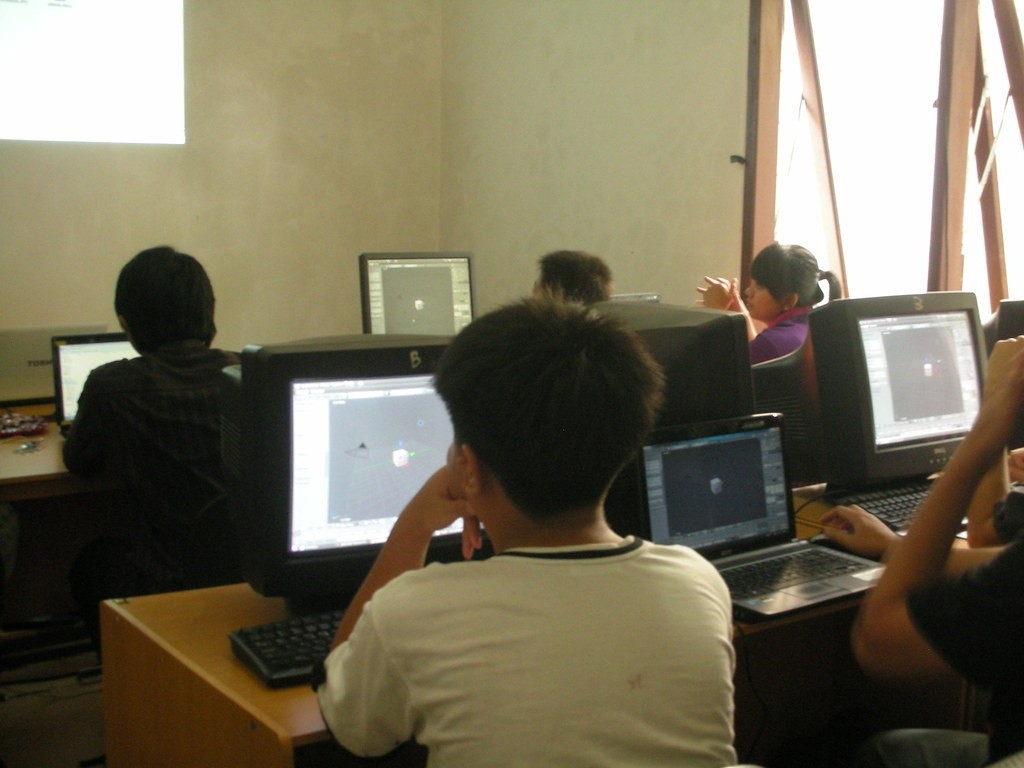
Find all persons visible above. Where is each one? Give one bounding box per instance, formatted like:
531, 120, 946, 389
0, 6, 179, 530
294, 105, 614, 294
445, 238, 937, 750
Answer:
317, 297, 737, 768
697, 244, 841, 366
63, 247, 245, 662
820, 334, 1024, 768
533, 250, 611, 307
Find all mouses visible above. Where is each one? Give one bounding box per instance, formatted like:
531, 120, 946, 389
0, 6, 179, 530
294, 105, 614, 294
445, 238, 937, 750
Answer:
809, 532, 882, 561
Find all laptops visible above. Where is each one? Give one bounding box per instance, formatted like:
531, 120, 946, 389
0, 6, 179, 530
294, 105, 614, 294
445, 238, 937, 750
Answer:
629, 412, 888, 622
52, 333, 143, 440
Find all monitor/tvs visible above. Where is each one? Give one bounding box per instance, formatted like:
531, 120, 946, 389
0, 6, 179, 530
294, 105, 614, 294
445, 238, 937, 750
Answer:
359, 252, 476, 338
243, 335, 495, 616
603, 291, 1024, 542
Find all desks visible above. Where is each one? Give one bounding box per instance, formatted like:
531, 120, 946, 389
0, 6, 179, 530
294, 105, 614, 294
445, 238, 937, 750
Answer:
99, 496, 868, 768
0, 404, 118, 503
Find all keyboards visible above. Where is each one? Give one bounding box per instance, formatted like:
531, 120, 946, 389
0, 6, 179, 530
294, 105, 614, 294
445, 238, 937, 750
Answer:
227, 612, 344, 689
833, 479, 970, 540
